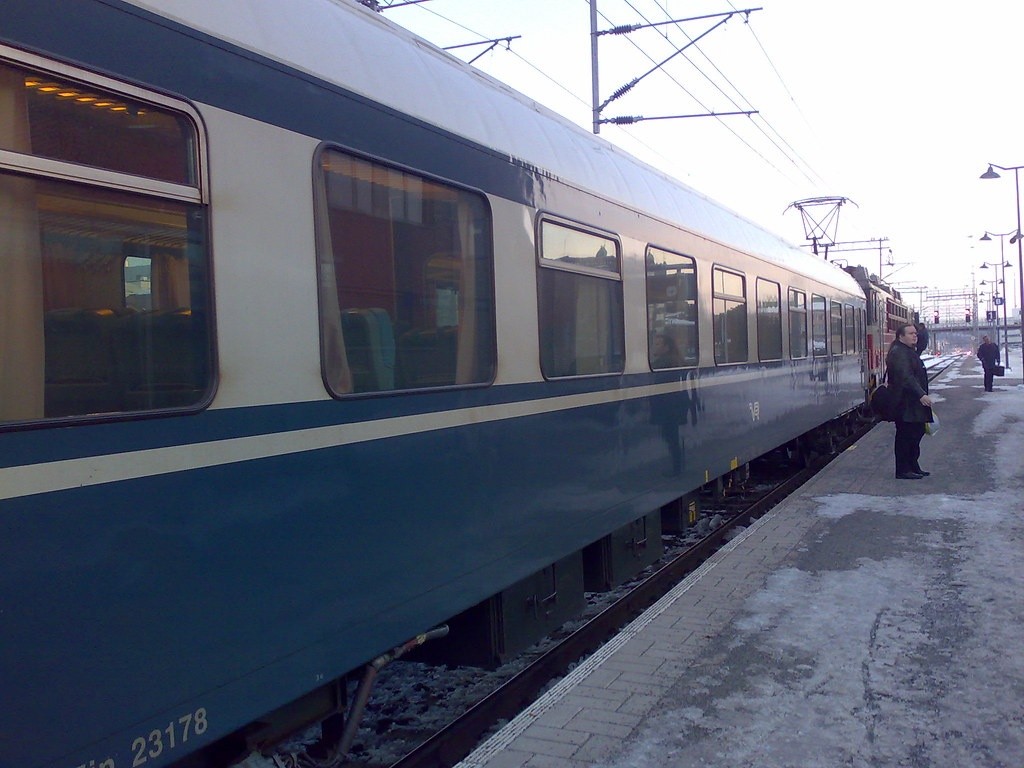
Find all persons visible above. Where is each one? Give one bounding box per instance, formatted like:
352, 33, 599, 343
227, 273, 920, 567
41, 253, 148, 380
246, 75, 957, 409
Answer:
978, 335, 1000, 391
914, 323, 928, 356
643, 337, 698, 494
886, 325, 931, 478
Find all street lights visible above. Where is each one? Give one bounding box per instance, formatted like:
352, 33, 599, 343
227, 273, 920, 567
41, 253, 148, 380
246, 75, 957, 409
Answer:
978, 162, 1024, 385
979, 228, 1023, 369
980, 279, 1005, 345
979, 260, 1013, 353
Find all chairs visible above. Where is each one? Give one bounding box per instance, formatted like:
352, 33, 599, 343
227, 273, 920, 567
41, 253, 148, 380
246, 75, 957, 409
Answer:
45, 298, 458, 418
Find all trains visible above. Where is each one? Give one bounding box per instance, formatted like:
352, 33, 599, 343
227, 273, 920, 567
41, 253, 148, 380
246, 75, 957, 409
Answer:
1, 1, 918, 767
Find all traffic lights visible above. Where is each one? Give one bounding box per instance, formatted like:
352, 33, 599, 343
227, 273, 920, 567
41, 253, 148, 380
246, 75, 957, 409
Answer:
934, 311, 939, 324
965, 309, 970, 322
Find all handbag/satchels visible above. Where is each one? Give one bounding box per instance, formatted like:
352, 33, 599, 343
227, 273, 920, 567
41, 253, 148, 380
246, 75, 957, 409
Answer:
867, 385, 895, 421
993, 364, 1005, 375
924, 410, 940, 435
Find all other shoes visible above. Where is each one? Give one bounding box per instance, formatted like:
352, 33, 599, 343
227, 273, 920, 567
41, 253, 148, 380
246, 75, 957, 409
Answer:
895, 471, 923, 479
913, 468, 930, 476
